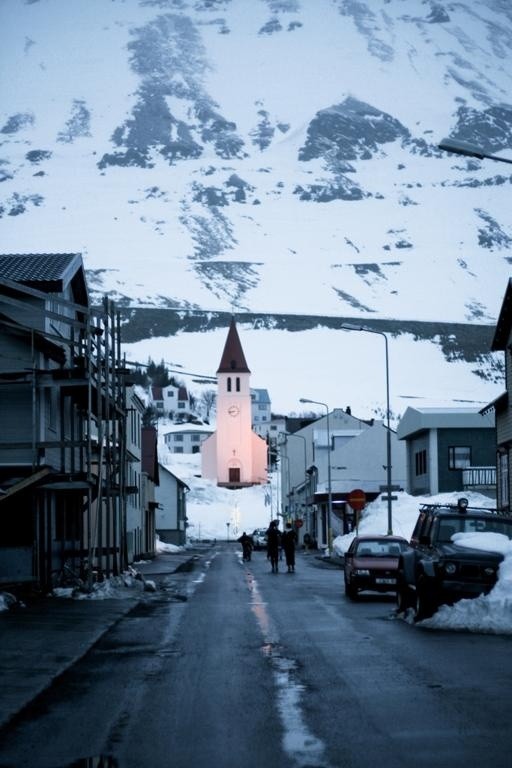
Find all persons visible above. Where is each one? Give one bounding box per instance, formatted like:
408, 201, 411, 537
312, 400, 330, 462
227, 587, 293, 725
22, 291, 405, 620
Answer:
265, 518, 296, 573
239, 529, 251, 561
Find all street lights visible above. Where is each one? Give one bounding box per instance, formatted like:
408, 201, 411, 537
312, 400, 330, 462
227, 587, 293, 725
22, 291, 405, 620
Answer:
340, 322, 392, 534
256, 397, 334, 557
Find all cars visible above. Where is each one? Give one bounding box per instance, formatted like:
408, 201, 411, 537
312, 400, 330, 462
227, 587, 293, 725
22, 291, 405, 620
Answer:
343, 536, 410, 601
252, 528, 267, 550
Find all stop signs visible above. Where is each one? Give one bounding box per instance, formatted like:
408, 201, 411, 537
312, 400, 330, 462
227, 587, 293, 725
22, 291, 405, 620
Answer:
348, 490, 368, 510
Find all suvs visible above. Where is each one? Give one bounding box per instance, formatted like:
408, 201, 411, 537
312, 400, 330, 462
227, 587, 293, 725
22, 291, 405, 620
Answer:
396, 498, 512, 624
